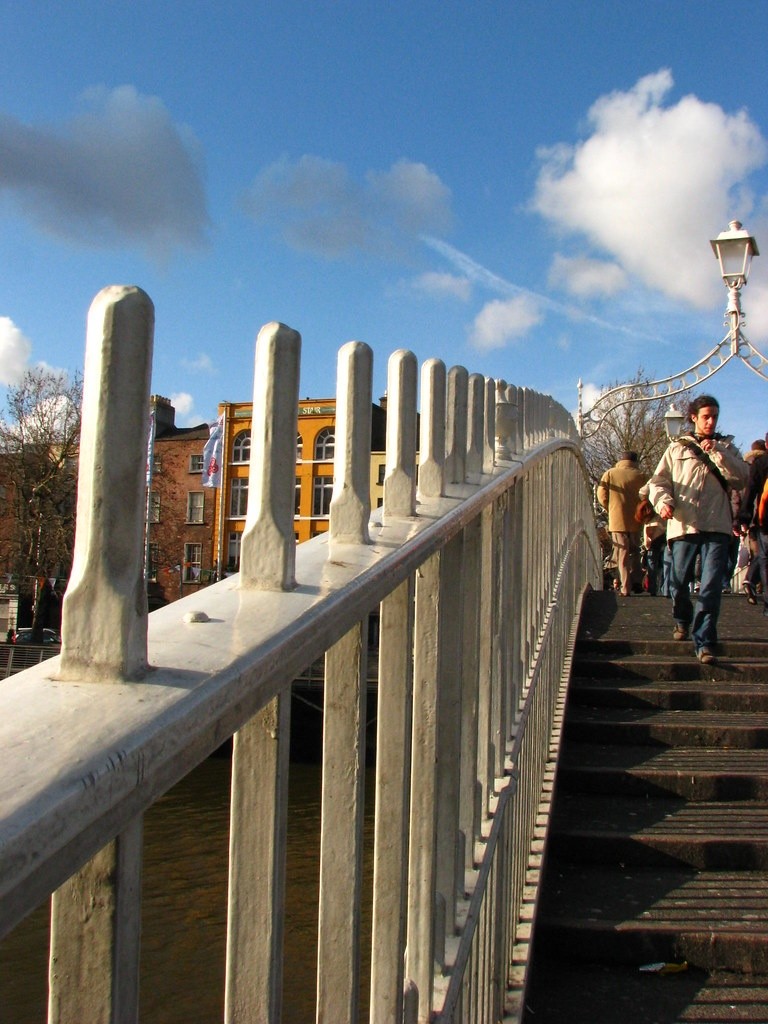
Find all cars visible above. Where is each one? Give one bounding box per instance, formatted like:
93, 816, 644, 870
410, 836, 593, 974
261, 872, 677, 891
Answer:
12, 627, 61, 645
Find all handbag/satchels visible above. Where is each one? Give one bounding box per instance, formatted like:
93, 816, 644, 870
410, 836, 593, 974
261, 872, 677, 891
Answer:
635, 496, 655, 526
729, 488, 749, 538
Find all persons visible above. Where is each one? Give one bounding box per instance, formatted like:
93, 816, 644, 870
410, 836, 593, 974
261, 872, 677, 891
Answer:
649, 396, 750, 664
597, 429, 768, 615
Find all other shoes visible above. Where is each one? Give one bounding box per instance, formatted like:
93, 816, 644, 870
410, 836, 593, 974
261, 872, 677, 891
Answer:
721, 586, 732, 595
763, 604, 768, 617
744, 582, 758, 606
695, 645, 716, 665
672, 624, 690, 640
